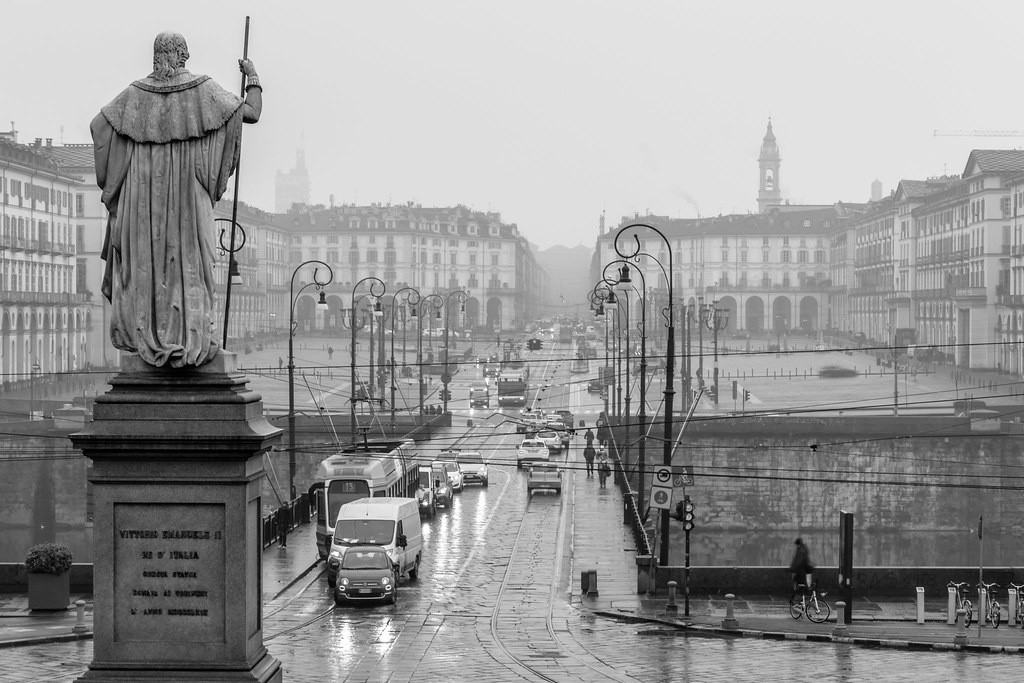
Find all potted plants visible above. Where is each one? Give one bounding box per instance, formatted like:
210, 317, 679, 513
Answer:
24, 544, 73, 610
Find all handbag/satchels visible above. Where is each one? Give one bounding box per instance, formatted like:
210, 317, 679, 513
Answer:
606, 466, 611, 476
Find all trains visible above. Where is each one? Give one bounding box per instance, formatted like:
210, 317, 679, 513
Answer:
316, 398, 419, 563
498, 348, 530, 405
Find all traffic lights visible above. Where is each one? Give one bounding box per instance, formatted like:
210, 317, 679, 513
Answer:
527, 339, 543, 350
746, 390, 750, 401
682, 502, 695, 532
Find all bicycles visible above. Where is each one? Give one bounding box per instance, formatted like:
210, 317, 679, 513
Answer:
789, 580, 831, 623
946, 580, 1024, 629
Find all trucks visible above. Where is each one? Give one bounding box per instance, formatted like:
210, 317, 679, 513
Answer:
328, 497, 424, 587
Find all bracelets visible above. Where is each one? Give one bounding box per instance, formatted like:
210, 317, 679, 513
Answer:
245, 78, 263, 92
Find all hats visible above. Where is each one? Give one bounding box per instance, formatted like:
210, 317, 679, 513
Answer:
793, 537, 804, 546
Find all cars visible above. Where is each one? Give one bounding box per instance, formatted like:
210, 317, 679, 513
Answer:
419, 449, 487, 520
469, 352, 499, 408
334, 546, 399, 606
525, 315, 598, 359
517, 408, 575, 494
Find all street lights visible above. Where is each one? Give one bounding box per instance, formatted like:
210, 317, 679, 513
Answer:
689, 297, 730, 403
390, 288, 420, 438
419, 295, 443, 426
348, 277, 386, 444
444, 291, 468, 414
603, 260, 645, 520
614, 224, 676, 567
289, 261, 334, 502
587, 280, 630, 474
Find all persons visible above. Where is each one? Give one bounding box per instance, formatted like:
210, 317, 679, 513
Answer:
595, 444, 607, 462
790, 537, 808, 592
277, 500, 290, 549
583, 442, 596, 476
597, 425, 606, 445
584, 428, 594, 447
89, 31, 263, 365
597, 453, 607, 488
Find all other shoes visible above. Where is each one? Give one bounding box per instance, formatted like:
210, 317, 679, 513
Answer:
587, 471, 593, 478
600, 483, 606, 489
